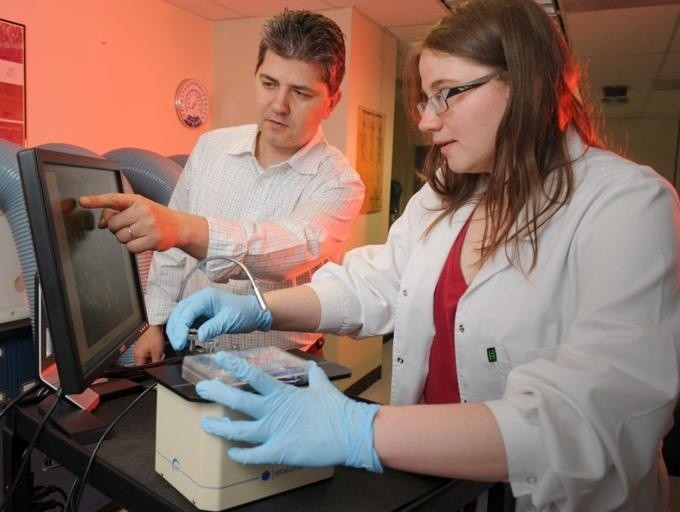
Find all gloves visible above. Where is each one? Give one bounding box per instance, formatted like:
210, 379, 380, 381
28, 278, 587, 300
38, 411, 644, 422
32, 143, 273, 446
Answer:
167, 288, 273, 351
194, 351, 386, 474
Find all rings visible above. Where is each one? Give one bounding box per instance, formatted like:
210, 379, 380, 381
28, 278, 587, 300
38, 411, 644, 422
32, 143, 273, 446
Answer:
128, 226, 136, 240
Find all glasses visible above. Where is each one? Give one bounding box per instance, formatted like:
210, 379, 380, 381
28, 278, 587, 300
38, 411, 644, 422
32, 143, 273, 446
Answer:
416, 70, 500, 117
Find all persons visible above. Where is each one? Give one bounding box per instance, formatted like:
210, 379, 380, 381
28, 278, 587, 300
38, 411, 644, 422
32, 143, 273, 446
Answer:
164, 0, 679, 512
78, 5, 366, 365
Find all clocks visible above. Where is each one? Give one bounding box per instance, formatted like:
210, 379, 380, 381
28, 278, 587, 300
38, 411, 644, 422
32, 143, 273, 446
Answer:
176, 79, 211, 129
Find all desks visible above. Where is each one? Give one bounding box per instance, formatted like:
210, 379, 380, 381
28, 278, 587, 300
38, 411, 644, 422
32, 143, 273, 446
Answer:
1, 385, 517, 512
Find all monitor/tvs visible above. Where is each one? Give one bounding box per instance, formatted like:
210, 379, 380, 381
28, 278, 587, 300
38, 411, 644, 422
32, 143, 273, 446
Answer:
16, 147, 151, 446
